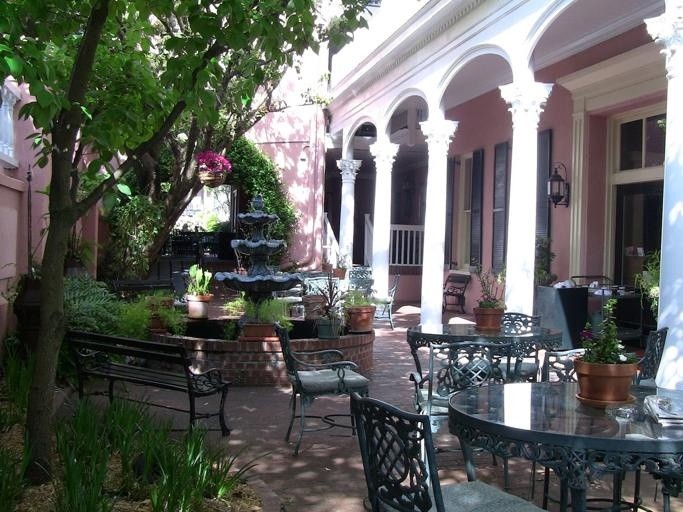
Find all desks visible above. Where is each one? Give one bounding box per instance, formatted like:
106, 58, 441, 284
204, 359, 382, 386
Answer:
446, 380, 683, 511
409, 322, 563, 386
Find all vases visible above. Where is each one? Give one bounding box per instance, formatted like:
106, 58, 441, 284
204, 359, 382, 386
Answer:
473, 308, 504, 328
575, 359, 635, 406
199, 173, 227, 188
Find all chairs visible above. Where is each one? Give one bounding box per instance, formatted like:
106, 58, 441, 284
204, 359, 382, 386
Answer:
306, 269, 401, 330
545, 327, 668, 387
350, 392, 547, 512
412, 342, 512, 467
492, 314, 541, 381
171, 229, 216, 257
274, 322, 370, 456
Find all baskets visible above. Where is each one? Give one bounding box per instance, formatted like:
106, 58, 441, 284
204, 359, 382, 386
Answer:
198, 168, 226, 188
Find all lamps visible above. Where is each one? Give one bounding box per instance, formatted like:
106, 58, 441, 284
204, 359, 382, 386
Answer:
547, 161, 569, 207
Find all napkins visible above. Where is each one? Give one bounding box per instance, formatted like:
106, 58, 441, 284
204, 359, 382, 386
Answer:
643, 395, 681, 426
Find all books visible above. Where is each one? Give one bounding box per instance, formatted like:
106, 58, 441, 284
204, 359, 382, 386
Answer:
644, 395, 683, 427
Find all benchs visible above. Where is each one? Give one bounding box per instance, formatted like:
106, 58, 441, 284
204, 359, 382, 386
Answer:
442, 273, 471, 313
110, 279, 175, 301
64, 329, 230, 444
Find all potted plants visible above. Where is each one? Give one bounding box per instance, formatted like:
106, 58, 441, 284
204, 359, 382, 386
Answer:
186, 264, 214, 319
227, 297, 294, 336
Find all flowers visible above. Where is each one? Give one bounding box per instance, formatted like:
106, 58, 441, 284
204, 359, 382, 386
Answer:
631, 250, 661, 318
582, 298, 641, 365
475, 286, 504, 308
196, 150, 232, 175
